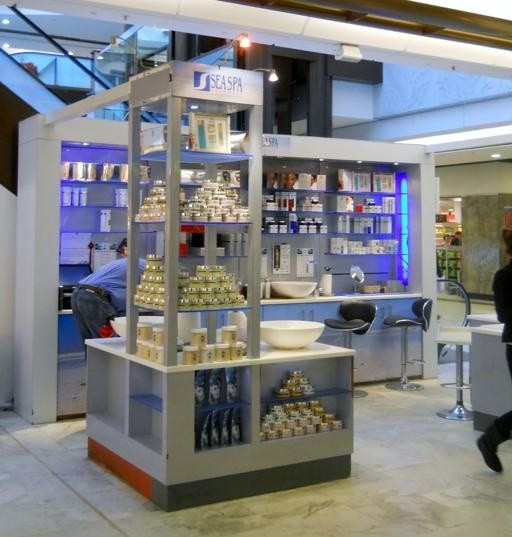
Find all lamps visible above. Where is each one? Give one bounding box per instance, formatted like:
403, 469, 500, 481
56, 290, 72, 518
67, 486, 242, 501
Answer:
253, 66, 280, 82
212, 31, 250, 66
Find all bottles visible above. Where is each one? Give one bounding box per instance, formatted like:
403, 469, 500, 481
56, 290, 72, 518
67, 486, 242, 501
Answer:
99, 209, 111, 232
228, 310, 248, 342
265, 278, 270, 298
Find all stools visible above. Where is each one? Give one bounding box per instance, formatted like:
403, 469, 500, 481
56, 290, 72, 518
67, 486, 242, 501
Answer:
437, 325, 476, 422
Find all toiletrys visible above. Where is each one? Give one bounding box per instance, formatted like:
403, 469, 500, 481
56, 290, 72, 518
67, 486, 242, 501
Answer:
60, 186, 91, 208
133, 253, 247, 365
59, 160, 151, 184
259, 368, 344, 439
99, 207, 111, 232
132, 160, 400, 255
113, 188, 145, 210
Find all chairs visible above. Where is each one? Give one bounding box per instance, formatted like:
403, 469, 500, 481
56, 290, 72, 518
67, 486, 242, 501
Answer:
383, 297, 435, 393
323, 301, 378, 398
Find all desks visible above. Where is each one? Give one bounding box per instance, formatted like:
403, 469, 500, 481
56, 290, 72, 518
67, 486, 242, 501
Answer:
464, 313, 512, 436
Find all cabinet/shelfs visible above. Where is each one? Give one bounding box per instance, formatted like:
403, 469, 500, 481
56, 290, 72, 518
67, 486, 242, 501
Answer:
82, 333, 356, 512
436, 223, 464, 296
126, 61, 263, 367
155, 310, 254, 332
192, 297, 427, 388
56, 351, 88, 418
261, 303, 349, 348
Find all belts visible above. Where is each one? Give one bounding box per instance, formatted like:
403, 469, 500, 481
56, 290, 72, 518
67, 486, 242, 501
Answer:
75, 283, 112, 303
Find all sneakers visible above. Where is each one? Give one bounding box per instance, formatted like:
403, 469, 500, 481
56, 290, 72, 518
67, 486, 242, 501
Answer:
477, 435, 503, 473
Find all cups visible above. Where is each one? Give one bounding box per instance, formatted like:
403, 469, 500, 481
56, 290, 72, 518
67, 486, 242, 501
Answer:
177, 312, 202, 344
320, 271, 332, 295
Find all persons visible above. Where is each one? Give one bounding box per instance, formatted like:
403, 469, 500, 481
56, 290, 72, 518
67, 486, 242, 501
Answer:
450, 231, 462, 245
474, 219, 511, 473
442, 234, 451, 244
71, 255, 148, 363
